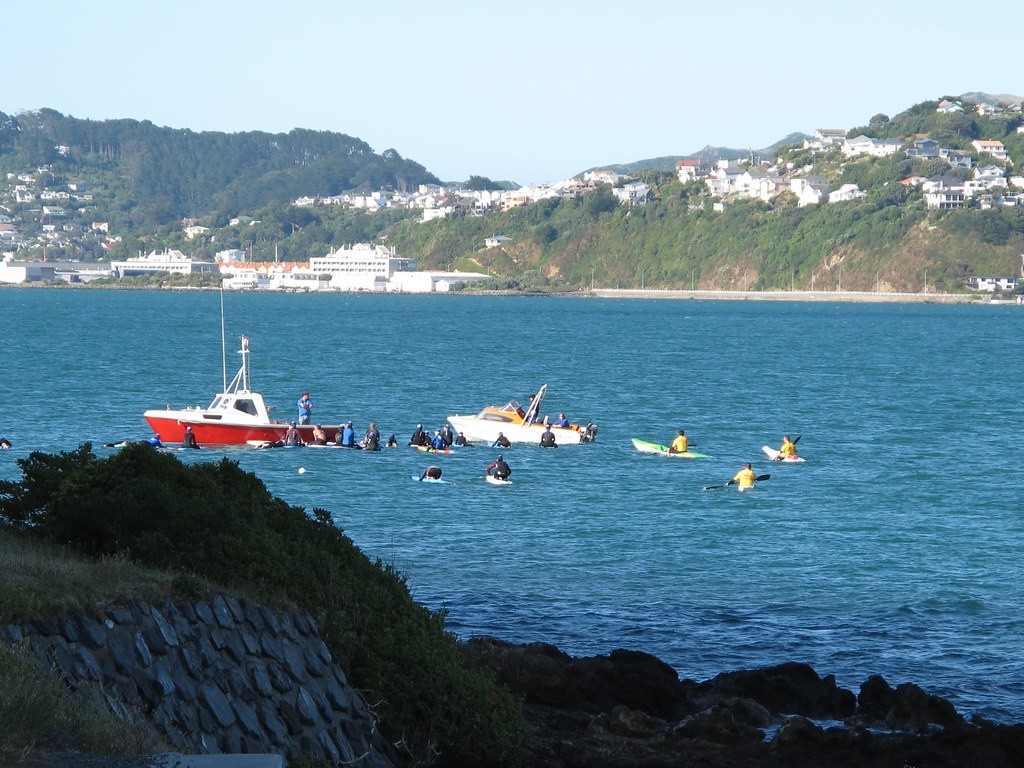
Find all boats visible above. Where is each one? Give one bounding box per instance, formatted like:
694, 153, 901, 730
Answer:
738, 483, 755, 492
762, 445, 805, 462
447, 383, 599, 445
418, 446, 458, 454
631, 438, 712, 458
142, 289, 347, 444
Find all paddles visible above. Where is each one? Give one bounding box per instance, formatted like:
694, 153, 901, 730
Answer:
775, 436, 802, 459
703, 474, 770, 490
660, 444, 699, 451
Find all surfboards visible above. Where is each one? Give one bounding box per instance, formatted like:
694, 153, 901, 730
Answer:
410, 440, 462, 453
762, 445, 806, 462
412, 476, 452, 484
486, 474, 514, 485
114, 441, 399, 449
488, 443, 505, 448
541, 446, 557, 449
739, 483, 755, 490
631, 439, 714, 458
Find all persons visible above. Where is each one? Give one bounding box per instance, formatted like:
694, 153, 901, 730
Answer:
486, 455, 512, 481
724, 463, 757, 489
774, 434, 798, 461
255, 393, 570, 450
296, 392, 314, 424
669, 429, 690, 453
182, 426, 201, 450
103, 433, 168, 448
418, 465, 443, 483
